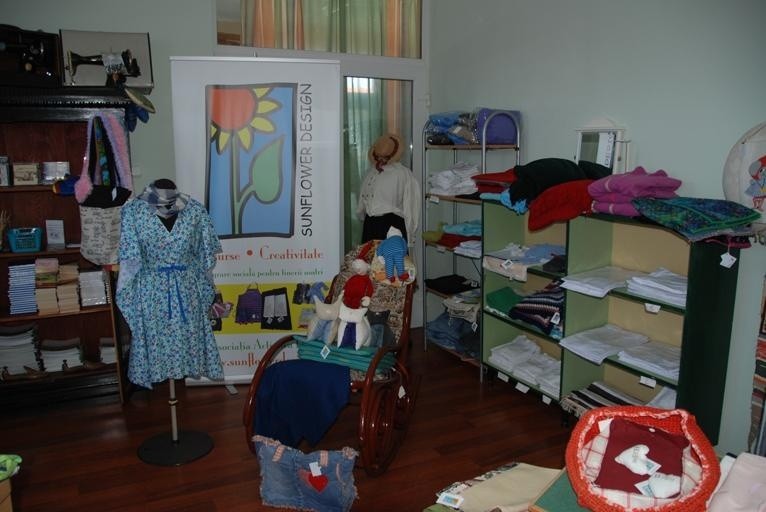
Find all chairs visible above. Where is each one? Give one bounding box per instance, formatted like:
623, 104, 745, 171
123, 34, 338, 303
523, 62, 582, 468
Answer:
240, 270, 426, 479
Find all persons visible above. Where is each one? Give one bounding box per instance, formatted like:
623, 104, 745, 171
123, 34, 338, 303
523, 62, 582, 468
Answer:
117, 179, 226, 388
355, 134, 422, 254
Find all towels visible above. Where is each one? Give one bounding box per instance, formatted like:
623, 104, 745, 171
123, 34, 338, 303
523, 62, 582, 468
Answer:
426, 273, 482, 365
558, 323, 680, 387
422, 105, 522, 147
559, 265, 690, 311
489, 333, 560, 403
426, 161, 483, 203
561, 380, 679, 420
423, 217, 484, 260
472, 148, 761, 284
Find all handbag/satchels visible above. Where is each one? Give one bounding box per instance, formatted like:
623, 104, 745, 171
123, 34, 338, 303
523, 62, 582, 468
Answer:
79, 186, 134, 267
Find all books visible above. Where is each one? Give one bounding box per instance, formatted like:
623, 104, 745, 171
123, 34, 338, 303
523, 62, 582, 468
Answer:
0, 256, 118, 377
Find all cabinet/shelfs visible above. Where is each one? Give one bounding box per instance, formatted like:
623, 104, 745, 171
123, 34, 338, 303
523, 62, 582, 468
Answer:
559, 215, 766, 459
0, 84, 138, 420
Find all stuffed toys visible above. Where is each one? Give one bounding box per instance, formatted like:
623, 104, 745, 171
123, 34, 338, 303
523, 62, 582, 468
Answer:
341, 241, 374, 307
365, 308, 396, 349
376, 226, 410, 282
337, 304, 371, 350
304, 293, 342, 345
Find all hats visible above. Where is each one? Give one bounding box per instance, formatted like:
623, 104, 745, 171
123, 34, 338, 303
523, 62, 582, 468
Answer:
368, 134, 404, 166
